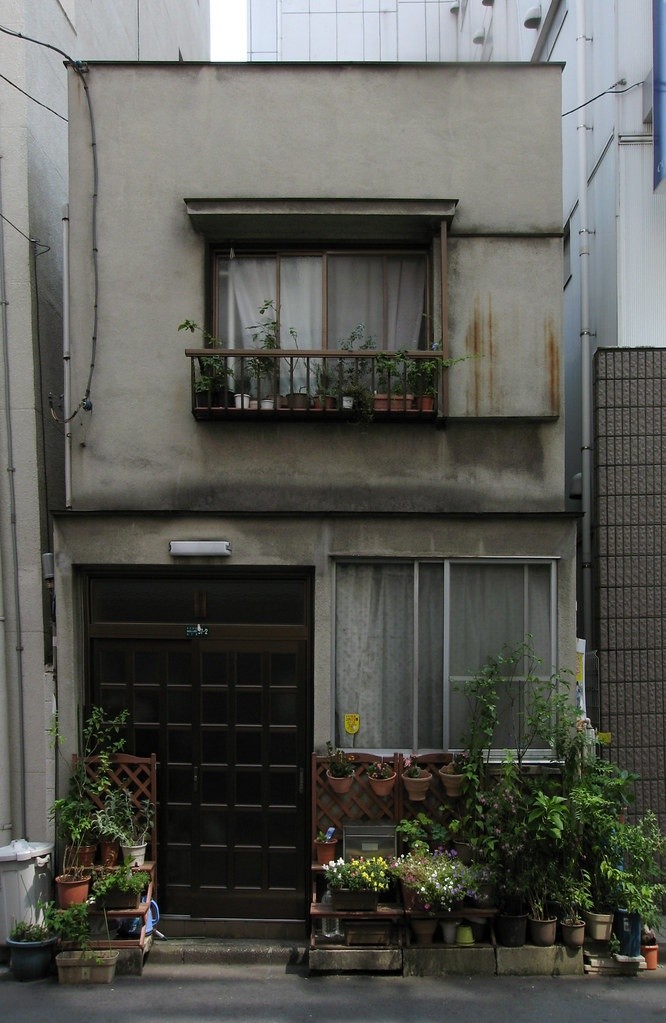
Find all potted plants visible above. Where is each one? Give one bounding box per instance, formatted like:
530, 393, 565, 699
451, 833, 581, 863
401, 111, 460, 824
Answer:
438, 629, 666, 970
6, 706, 153, 986
178, 299, 485, 434
313, 831, 337, 865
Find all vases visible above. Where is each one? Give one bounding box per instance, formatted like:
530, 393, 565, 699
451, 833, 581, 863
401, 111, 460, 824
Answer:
401, 773, 433, 801
368, 772, 397, 796
329, 887, 473, 946
326, 769, 353, 794
439, 769, 464, 797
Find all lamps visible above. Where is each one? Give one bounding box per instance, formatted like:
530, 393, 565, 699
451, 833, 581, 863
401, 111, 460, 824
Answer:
169, 540, 232, 557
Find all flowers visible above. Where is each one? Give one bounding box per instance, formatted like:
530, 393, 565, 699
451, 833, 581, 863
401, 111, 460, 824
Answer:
319, 740, 522, 911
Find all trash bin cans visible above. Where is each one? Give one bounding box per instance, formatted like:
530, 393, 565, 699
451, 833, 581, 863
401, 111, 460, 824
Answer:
0, 838, 55, 946
